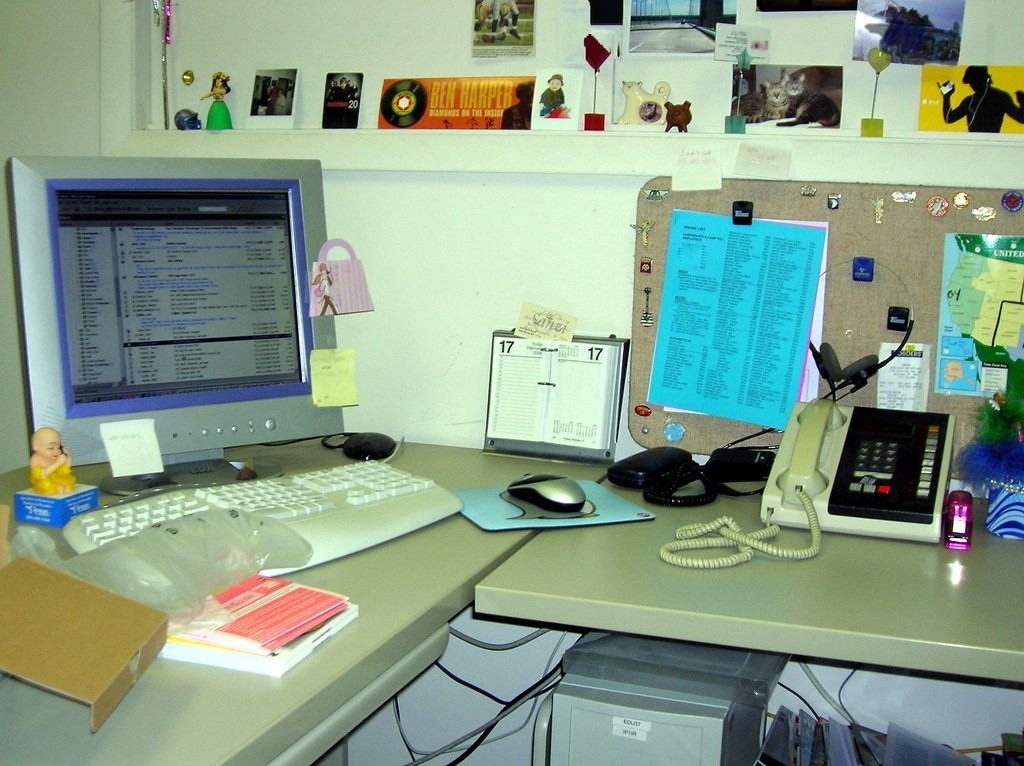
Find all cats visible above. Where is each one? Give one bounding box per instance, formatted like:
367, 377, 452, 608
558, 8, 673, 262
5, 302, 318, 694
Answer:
731, 72, 840, 126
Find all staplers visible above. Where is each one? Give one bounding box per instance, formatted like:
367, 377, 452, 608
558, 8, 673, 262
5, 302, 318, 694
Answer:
945, 489, 975, 552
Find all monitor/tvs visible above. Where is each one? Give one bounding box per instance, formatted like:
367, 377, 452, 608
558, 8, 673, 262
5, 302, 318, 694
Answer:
9, 156, 346, 496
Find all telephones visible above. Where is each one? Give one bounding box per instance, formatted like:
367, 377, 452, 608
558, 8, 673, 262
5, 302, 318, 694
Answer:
758, 394, 960, 546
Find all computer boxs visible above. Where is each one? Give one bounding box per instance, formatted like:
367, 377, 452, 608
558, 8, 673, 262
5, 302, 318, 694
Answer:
550, 669, 770, 765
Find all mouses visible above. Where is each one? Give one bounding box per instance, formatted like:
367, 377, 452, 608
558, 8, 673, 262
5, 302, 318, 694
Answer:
507, 473, 588, 513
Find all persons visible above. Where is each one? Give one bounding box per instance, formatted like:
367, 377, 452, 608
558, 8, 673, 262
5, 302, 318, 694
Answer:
256, 84, 286, 115
474, 0, 522, 44
538, 74, 566, 119
28, 427, 76, 495
327, 75, 359, 126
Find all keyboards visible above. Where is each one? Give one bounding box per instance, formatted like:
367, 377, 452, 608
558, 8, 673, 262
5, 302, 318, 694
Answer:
63, 457, 463, 585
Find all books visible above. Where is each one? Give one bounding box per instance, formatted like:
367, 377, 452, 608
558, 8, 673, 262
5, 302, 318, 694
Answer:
158, 574, 359, 678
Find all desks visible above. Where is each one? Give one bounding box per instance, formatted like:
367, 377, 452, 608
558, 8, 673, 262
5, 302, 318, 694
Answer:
471, 474, 1023, 765
0, 436, 614, 766
